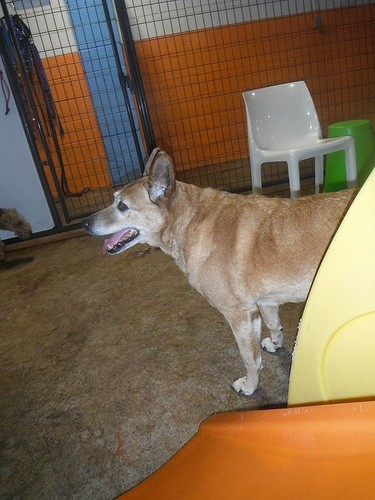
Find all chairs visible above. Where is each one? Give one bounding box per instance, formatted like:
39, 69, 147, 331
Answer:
240, 82, 359, 202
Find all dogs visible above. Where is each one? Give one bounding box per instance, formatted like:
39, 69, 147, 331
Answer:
82, 148, 360, 397
0, 207, 32, 262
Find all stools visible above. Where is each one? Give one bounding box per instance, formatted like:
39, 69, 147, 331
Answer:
322, 118, 375, 192
